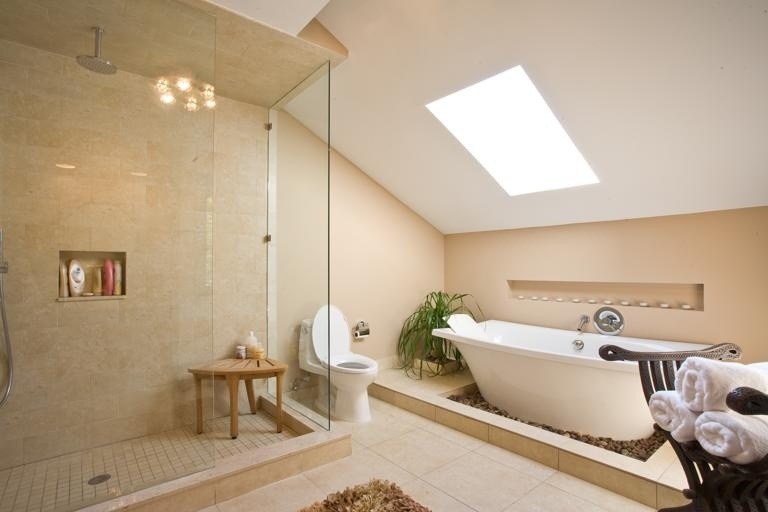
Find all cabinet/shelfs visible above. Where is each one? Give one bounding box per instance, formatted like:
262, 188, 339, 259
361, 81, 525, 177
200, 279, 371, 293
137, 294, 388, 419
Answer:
597, 344, 768, 511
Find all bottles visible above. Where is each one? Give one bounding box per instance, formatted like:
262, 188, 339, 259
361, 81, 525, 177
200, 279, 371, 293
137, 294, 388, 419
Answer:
235, 330, 264, 361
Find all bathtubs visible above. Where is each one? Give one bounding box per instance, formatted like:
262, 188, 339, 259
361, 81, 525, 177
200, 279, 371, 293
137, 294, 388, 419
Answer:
431, 319, 714, 442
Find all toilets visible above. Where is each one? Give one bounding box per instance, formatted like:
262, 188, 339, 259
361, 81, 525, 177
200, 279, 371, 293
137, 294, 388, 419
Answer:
299, 304, 379, 425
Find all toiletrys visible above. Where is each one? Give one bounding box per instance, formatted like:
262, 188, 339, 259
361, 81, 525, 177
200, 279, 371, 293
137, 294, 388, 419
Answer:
69, 258, 85, 296
236, 331, 266, 360
113, 260, 123, 295
104, 259, 113, 296
59, 263, 69, 298
93, 268, 103, 295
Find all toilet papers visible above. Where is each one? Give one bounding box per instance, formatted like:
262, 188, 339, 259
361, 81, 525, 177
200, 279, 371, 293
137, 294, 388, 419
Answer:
354, 329, 369, 340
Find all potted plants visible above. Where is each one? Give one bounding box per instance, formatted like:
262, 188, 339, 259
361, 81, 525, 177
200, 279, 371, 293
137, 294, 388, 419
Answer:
398, 290, 484, 381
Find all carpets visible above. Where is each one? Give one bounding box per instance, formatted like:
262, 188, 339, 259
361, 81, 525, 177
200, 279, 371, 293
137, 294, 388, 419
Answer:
299, 478, 430, 512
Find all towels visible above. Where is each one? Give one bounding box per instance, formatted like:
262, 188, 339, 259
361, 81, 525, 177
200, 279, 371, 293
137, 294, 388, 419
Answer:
648, 355, 768, 465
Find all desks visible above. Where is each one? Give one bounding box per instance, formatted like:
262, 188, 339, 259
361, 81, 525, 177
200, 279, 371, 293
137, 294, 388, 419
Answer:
189, 358, 290, 440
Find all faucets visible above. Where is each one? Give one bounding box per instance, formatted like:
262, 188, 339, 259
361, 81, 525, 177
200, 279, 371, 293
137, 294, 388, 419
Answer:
577, 314, 590, 333
